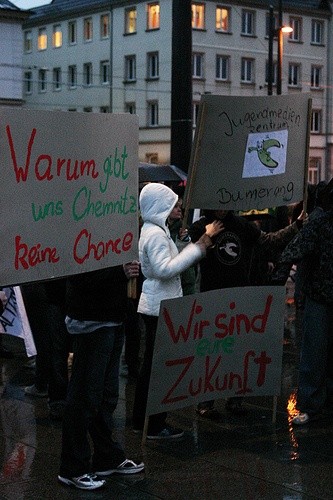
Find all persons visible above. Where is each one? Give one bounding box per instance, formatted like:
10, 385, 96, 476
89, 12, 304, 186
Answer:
166, 196, 196, 296
273, 186, 333, 423
189, 209, 309, 416
58, 260, 145, 488
136, 183, 224, 439
25, 281, 74, 427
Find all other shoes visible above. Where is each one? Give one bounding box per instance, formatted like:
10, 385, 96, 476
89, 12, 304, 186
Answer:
291, 408, 309, 425
25, 359, 36, 368
224, 399, 247, 413
193, 406, 219, 419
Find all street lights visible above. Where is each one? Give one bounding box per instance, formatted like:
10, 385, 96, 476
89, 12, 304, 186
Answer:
276, 25, 295, 95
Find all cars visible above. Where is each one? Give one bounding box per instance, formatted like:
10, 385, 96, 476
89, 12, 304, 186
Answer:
138, 164, 188, 195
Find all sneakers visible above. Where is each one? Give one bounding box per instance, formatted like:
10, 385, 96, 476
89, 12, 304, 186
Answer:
25, 384, 48, 396
133, 404, 147, 424
58, 473, 106, 490
95, 455, 144, 476
146, 423, 184, 439
